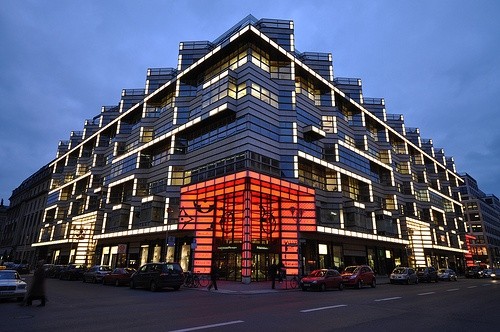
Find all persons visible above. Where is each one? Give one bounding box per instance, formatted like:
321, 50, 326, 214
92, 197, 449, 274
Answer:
207, 261, 219, 290
25, 260, 47, 307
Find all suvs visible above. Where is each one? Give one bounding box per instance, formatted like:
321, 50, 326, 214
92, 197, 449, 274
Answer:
128, 261, 185, 291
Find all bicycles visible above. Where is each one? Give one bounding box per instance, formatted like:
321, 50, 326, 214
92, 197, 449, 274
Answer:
184, 270, 210, 288
290, 274, 300, 289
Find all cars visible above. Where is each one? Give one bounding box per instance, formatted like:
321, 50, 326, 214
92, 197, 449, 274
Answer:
465, 265, 500, 280
389, 267, 417, 285
436, 268, 458, 281
299, 268, 344, 292
340, 265, 377, 289
0, 266, 28, 303
416, 267, 439, 283
33, 263, 87, 281
2, 262, 30, 275
103, 268, 137, 286
82, 265, 112, 284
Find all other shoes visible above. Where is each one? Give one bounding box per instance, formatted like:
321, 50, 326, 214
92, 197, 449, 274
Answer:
17, 303, 24, 306
207, 286, 210, 291
26, 304, 32, 307
37, 304, 45, 307
